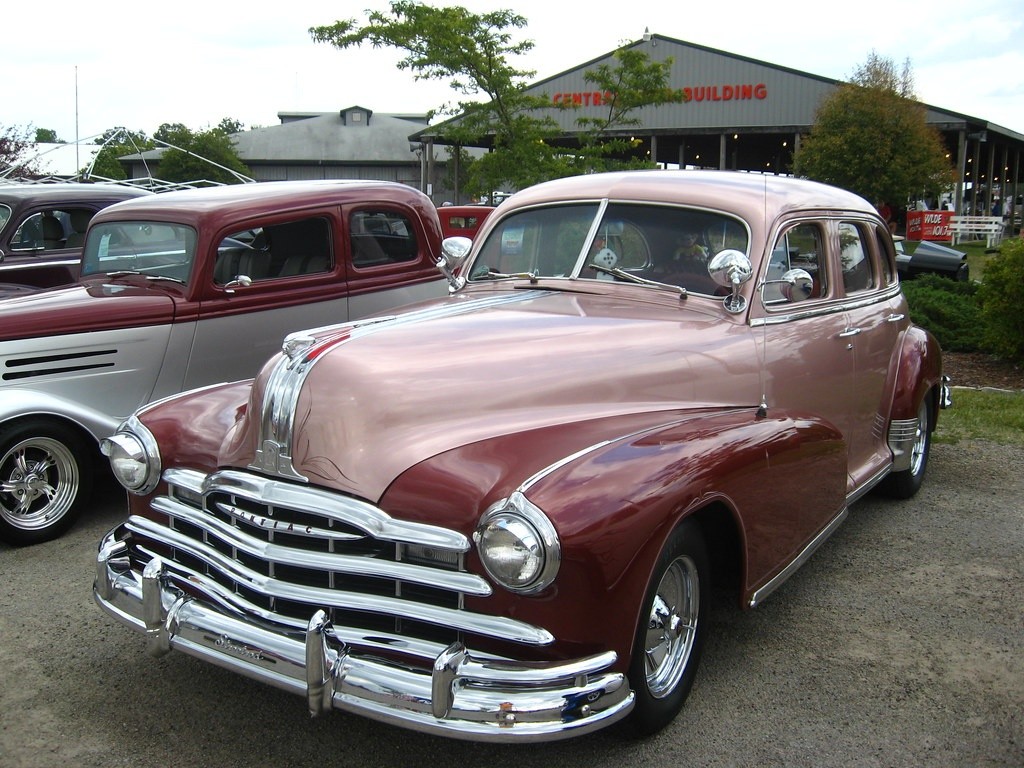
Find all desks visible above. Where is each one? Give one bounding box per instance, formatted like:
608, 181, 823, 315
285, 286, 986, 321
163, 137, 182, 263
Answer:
907, 210, 955, 241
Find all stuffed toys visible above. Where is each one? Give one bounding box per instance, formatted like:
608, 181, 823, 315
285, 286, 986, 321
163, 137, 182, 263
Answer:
672, 231, 708, 261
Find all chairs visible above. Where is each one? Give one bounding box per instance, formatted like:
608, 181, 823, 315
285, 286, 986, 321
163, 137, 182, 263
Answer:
660, 273, 729, 297
214, 248, 329, 284
34, 210, 93, 249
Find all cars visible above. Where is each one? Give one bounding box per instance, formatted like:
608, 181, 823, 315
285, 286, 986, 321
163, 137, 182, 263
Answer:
435, 204, 494, 278
1, 178, 451, 547
88, 168, 952, 745
771, 222, 970, 288
0, 173, 277, 306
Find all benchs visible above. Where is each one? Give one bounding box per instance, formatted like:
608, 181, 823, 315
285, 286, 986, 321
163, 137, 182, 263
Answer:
351, 235, 412, 267
946, 216, 1003, 248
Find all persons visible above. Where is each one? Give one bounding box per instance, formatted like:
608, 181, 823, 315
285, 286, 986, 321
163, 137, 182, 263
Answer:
876, 199, 897, 234
994, 198, 1010, 221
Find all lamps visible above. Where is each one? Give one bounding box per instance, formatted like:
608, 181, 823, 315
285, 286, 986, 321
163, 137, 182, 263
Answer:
643, 27, 656, 46
